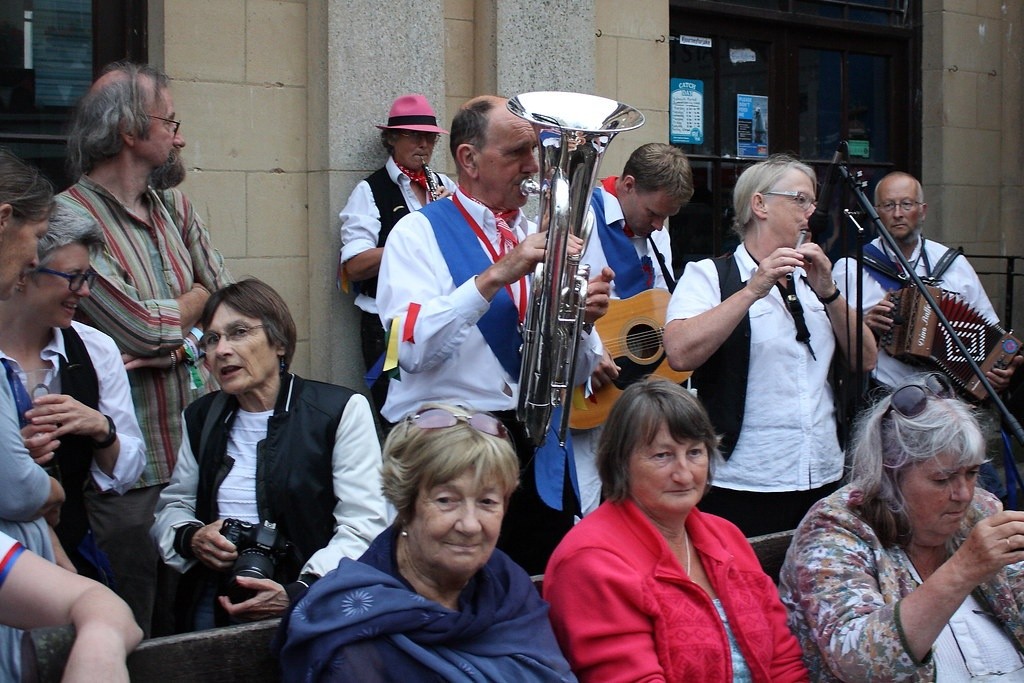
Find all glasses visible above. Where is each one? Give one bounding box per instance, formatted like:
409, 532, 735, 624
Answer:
883, 373, 956, 419
875, 197, 922, 212
146, 114, 181, 136
405, 408, 517, 454
397, 129, 441, 142
35, 266, 98, 292
762, 189, 818, 211
197, 324, 269, 353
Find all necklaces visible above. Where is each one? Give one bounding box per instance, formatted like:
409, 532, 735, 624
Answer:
685, 531, 691, 578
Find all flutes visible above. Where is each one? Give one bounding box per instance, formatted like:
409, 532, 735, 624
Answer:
418, 155, 442, 201
785, 230, 807, 281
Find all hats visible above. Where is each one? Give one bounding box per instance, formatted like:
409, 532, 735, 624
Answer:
375, 94, 450, 135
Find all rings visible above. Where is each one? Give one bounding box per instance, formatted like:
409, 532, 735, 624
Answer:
1006, 537, 1011, 549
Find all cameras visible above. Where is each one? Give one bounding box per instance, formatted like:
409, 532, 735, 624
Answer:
219, 517, 293, 605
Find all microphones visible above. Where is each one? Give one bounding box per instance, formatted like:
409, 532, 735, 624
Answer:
807, 140, 846, 234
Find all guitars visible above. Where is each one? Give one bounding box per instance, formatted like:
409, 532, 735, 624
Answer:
567, 288, 694, 430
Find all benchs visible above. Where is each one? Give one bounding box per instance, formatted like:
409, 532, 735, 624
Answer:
127, 531, 797, 683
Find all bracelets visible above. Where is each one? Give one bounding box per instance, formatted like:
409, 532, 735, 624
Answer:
168, 326, 211, 390
92, 414, 117, 448
819, 288, 841, 305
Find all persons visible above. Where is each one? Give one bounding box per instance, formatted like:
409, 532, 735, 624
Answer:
552, 143, 695, 534
830, 170, 1023, 441
37, 61, 237, 641
268, 403, 578, 683
374, 95, 616, 601
0, 530, 145, 682
149, 279, 398, 622
778, 372, 1024, 683
0, 148, 79, 683
545, 377, 809, 683
337, 94, 456, 438
1, 194, 147, 584
662, 154, 878, 584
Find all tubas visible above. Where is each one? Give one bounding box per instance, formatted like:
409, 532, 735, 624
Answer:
505, 89, 645, 448
